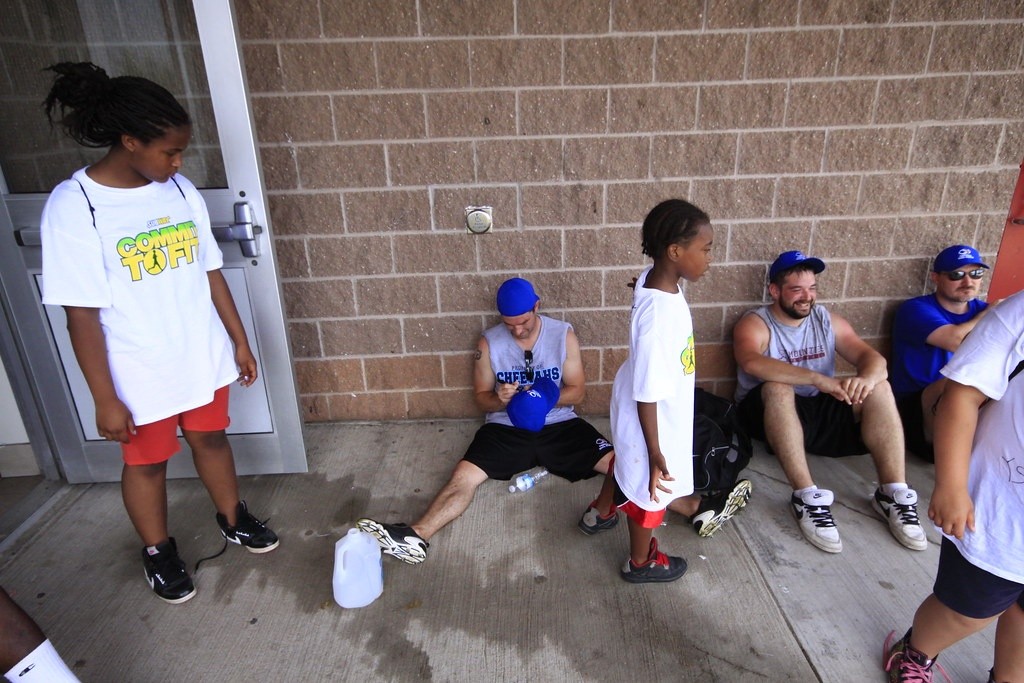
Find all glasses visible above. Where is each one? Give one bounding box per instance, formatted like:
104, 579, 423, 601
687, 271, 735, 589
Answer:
523, 350, 535, 383
942, 268, 984, 281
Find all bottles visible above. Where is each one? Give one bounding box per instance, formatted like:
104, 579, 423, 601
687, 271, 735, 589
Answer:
332, 527, 384, 609
508, 465, 550, 494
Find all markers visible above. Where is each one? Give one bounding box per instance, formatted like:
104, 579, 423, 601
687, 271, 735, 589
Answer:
498, 380, 522, 391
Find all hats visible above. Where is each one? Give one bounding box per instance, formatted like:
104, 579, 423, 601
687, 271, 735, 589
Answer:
497, 277, 540, 316
506, 377, 560, 432
934, 244, 991, 272
768, 251, 825, 283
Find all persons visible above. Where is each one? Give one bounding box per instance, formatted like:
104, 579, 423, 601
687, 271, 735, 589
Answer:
0, 585, 82, 683
883, 289, 1024, 683
40, 63, 279, 604
354, 278, 752, 564
576, 198, 713, 583
889, 244, 1024, 463
732, 250, 928, 554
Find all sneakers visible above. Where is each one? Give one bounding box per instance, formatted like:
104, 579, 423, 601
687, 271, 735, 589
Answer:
690, 478, 752, 539
578, 501, 620, 535
216, 500, 278, 553
987, 667, 996, 683
141, 537, 196, 604
869, 482, 928, 552
621, 537, 687, 583
355, 518, 430, 565
791, 486, 843, 553
882, 626, 939, 683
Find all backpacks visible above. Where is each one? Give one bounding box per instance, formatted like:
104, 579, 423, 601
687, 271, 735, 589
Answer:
692, 386, 753, 493
